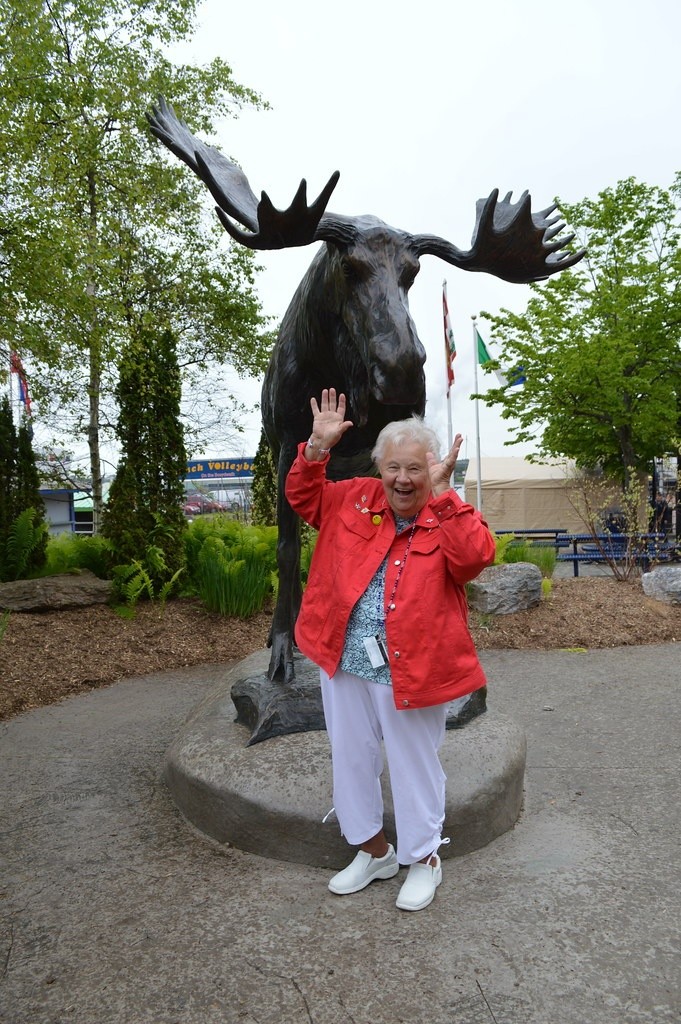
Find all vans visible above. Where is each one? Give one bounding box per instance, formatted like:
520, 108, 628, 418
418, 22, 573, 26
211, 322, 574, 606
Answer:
205, 490, 246, 512
187, 494, 227, 513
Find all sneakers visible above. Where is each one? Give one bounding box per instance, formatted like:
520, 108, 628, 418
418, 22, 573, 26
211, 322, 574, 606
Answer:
328, 843, 399, 896
395, 853, 443, 912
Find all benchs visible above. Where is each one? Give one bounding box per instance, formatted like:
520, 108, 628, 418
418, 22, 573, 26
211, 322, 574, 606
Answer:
582, 542, 681, 550
555, 552, 666, 577
510, 541, 570, 547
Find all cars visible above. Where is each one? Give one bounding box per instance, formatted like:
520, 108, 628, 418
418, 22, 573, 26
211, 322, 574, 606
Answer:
183, 503, 200, 515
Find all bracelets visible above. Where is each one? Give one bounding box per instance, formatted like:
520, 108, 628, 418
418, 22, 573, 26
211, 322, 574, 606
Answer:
308, 439, 330, 455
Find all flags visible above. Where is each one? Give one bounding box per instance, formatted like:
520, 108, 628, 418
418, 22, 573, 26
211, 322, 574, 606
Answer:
11, 346, 31, 416
443, 294, 457, 398
476, 328, 526, 386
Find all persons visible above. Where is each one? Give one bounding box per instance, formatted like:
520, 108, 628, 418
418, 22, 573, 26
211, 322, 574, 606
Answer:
284, 388, 496, 915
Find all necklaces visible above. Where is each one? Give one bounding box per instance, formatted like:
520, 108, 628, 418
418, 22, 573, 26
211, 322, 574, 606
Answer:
376, 510, 418, 636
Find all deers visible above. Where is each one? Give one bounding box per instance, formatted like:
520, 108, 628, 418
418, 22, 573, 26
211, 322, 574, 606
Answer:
136, 92, 589, 682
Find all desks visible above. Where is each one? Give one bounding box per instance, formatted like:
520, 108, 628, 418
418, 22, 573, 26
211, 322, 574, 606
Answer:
556, 534, 665, 573
495, 529, 568, 553
